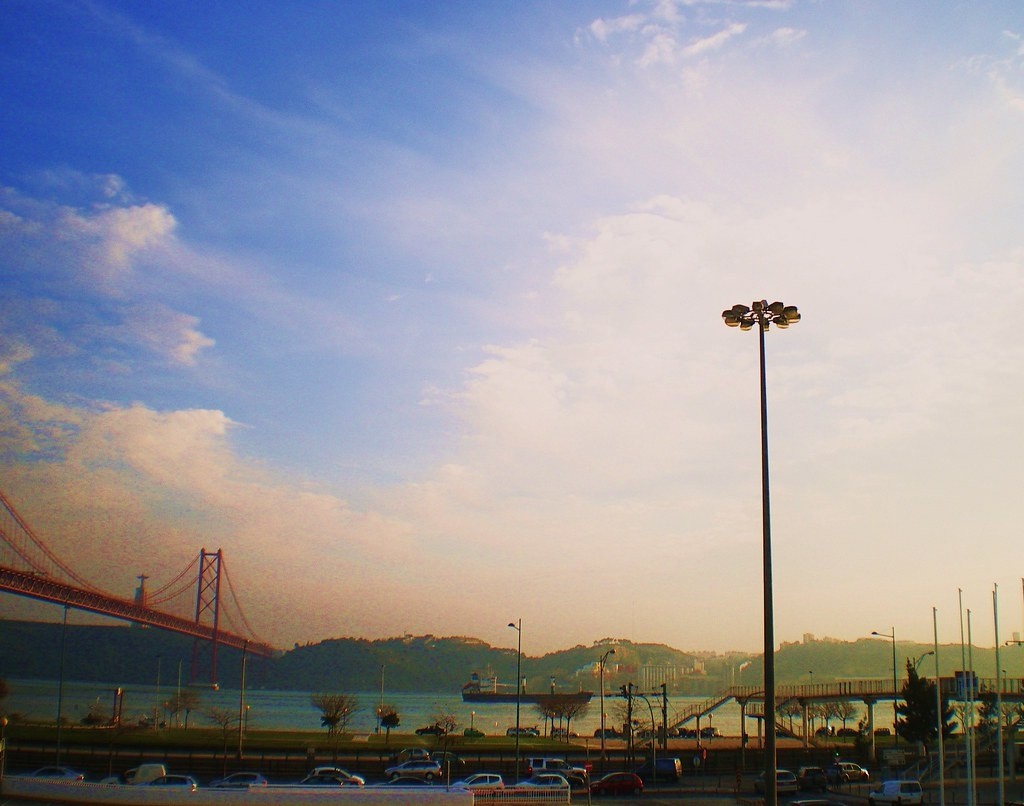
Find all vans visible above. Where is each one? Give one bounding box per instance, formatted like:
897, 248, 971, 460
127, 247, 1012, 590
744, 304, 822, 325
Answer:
629, 757, 682, 783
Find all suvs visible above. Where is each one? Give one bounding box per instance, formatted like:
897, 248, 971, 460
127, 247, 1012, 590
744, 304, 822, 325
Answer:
869, 781, 925, 806
797, 766, 827, 793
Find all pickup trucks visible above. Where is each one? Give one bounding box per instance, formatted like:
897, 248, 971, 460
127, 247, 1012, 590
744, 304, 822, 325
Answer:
101, 765, 167, 788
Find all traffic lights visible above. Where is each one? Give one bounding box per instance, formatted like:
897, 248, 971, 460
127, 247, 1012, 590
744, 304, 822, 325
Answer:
645, 741, 654, 769
619, 685, 627, 700
835, 745, 841, 759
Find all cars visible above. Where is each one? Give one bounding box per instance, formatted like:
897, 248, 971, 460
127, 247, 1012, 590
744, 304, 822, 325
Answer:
551, 728, 580, 739
299, 768, 366, 786
830, 763, 871, 786
413, 724, 446, 735
813, 725, 890, 738
15, 766, 87, 784
752, 769, 798, 796
669, 726, 720, 740
370, 748, 592, 801
209, 773, 268, 789
464, 728, 487, 738
136, 774, 199, 790
587, 772, 644, 798
507, 726, 540, 739
595, 728, 616, 739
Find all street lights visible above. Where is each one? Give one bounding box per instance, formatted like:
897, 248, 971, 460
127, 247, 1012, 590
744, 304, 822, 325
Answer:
600, 648, 617, 776
873, 630, 900, 747
470, 710, 475, 731
551, 711, 557, 739
377, 708, 381, 737
603, 712, 608, 732
508, 618, 522, 786
235, 640, 250, 759
54, 602, 74, 767
708, 713, 712, 744
721, 298, 803, 799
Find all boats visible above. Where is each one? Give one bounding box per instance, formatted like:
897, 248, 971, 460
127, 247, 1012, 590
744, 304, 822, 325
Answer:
461, 672, 594, 707
